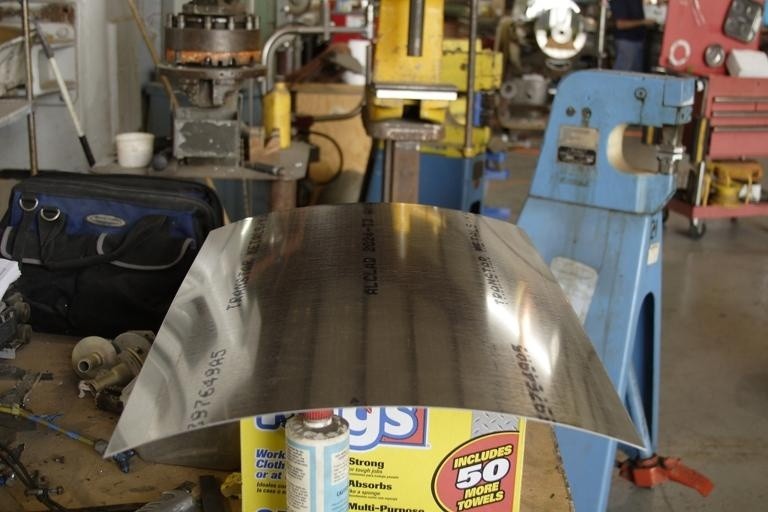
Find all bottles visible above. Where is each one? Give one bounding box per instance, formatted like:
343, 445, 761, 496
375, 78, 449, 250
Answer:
284, 409, 350, 512
262, 75, 292, 147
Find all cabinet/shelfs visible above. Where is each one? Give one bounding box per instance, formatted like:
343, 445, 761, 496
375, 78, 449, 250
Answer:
0, 0, 38, 177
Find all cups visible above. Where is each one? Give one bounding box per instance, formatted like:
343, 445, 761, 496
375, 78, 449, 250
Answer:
115, 132, 155, 170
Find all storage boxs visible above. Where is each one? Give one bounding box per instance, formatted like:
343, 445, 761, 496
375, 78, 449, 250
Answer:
238, 403, 526, 512
